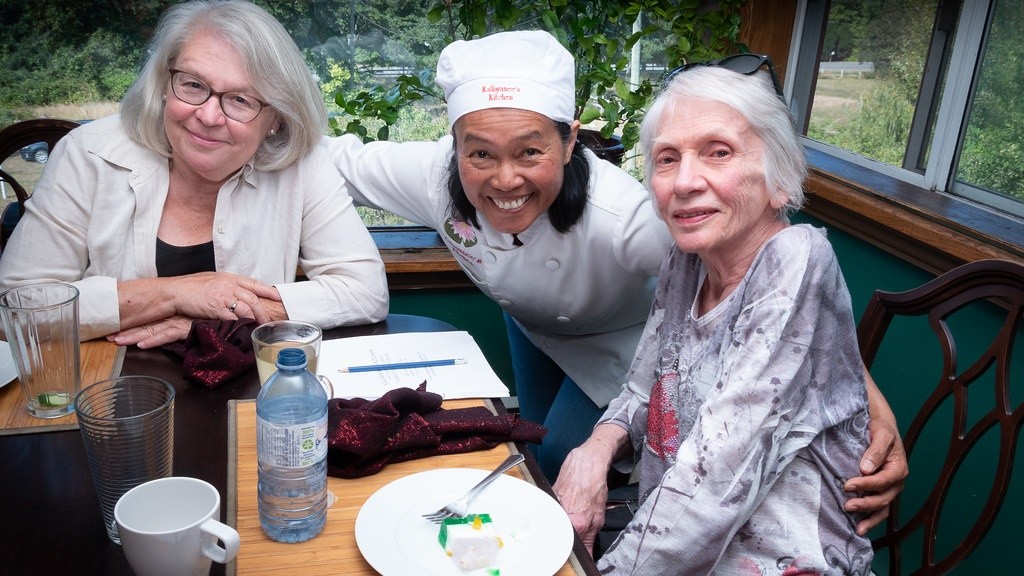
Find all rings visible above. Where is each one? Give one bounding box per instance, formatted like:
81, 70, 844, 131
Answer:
230, 301, 237, 312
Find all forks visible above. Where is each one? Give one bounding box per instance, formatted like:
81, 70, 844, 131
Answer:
422, 454, 525, 525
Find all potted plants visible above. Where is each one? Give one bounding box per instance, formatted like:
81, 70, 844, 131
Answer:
331, 0, 740, 175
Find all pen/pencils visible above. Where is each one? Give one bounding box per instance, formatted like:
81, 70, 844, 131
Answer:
336, 359, 468, 373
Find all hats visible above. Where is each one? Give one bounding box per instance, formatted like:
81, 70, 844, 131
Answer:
435, 30, 575, 127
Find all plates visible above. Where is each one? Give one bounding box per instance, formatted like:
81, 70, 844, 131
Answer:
355, 467, 574, 576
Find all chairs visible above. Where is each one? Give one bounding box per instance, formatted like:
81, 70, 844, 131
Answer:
851, 259, 1024, 576
0, 117, 86, 250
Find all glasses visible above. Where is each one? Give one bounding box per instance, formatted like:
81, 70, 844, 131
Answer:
664, 53, 784, 101
169, 68, 270, 123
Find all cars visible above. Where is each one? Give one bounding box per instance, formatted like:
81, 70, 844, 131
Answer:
19, 117, 95, 163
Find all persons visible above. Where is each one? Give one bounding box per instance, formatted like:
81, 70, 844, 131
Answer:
0, 0, 389, 348
315, 31, 909, 536
551, 53, 876, 576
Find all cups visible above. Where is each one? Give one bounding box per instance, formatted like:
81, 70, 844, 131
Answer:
75, 375, 176, 546
115, 477, 240, 575
252, 320, 333, 402
0, 283, 79, 419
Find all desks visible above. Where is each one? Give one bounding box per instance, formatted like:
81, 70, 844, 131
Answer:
1, 314, 599, 574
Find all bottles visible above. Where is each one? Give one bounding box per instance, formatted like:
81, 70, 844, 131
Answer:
255, 349, 328, 544
